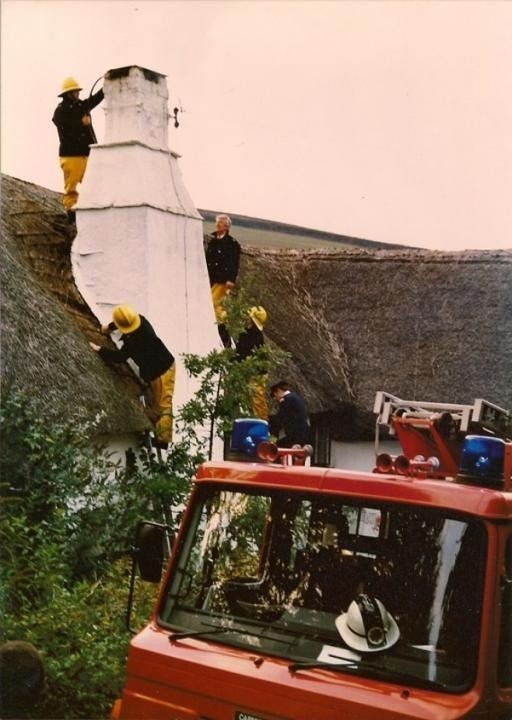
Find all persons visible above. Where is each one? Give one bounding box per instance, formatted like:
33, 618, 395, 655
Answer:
204, 214, 244, 347
231, 303, 272, 423
262, 379, 310, 451
51, 75, 105, 219
87, 305, 177, 448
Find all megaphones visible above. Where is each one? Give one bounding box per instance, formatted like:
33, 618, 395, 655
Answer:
256, 440, 314, 460
374, 446, 441, 475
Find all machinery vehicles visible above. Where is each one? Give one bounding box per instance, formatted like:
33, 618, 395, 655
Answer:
122, 387, 512, 720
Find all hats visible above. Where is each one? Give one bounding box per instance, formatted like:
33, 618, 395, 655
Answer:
269, 380, 289, 398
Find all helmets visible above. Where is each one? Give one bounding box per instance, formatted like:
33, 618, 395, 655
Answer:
112, 305, 141, 334
57, 77, 83, 96
247, 306, 267, 332
335, 594, 400, 652
